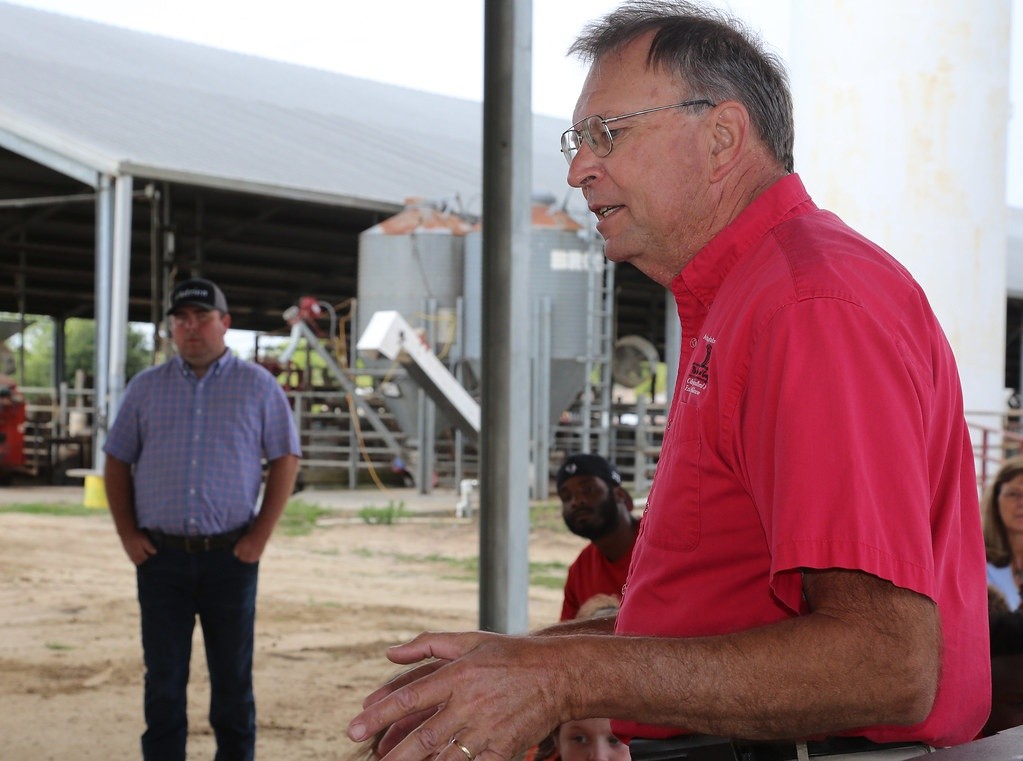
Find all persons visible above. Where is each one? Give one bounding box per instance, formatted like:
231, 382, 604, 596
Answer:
556, 454, 643, 622
101, 278, 303, 761
534, 717, 631, 761
982, 455, 1023, 741
348, 0, 993, 761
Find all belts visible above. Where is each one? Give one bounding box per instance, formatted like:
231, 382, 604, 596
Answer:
148, 527, 249, 553
629, 736, 922, 760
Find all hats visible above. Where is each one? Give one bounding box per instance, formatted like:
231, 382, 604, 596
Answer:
167, 279, 227, 313
556, 455, 633, 512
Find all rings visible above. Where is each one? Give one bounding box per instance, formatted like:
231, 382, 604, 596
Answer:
452, 739, 474, 761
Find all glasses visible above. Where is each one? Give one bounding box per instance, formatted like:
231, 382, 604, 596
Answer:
559, 100, 716, 166
